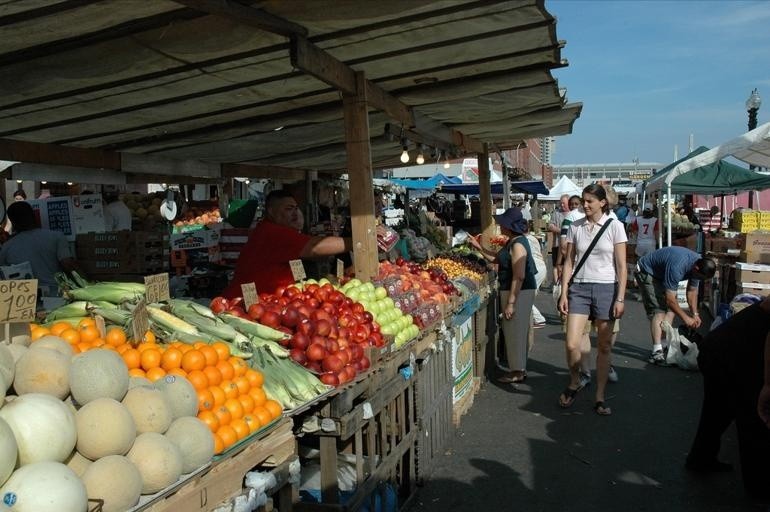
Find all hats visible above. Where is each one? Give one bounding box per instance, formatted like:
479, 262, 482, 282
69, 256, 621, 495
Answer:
491, 208, 527, 233
644, 203, 654, 211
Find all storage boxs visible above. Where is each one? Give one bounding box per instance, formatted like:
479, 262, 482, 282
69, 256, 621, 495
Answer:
23, 196, 71, 237
688, 237, 711, 251
77, 231, 164, 282
713, 239, 736, 253
677, 294, 689, 308
449, 316, 473, 381
735, 280, 770, 297
70, 194, 105, 235
627, 243, 637, 255
462, 159, 494, 182
626, 255, 637, 264
738, 232, 770, 264
220, 229, 250, 269
735, 263, 770, 284
699, 210, 722, 234
452, 364, 475, 429
678, 281, 689, 294
170, 229, 218, 252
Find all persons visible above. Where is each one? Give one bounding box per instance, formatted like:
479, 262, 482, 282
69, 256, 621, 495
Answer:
453, 184, 717, 416
229, 190, 387, 301
0, 189, 74, 298
107, 193, 133, 232
342, 195, 384, 251
682, 297, 768, 503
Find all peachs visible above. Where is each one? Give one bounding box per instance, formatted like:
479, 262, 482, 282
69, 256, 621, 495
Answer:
175, 206, 221, 225
382, 259, 448, 305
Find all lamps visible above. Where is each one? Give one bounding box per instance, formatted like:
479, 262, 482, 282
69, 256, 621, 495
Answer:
415, 150, 425, 165
444, 153, 451, 169
401, 146, 410, 163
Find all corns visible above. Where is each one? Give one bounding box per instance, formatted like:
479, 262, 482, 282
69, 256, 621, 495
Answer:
45, 280, 328, 412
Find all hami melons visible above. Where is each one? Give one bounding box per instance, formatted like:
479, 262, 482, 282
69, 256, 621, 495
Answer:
124, 191, 165, 222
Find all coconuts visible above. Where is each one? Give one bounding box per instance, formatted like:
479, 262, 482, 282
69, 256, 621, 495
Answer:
0, 336, 214, 510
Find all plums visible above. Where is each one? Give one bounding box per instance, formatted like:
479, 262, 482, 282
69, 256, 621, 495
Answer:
396, 256, 458, 296
211, 284, 385, 393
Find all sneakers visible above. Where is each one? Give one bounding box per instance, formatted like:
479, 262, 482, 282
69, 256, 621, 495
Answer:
496, 370, 528, 383
580, 372, 591, 386
532, 322, 546, 329
648, 350, 671, 367
596, 356, 618, 382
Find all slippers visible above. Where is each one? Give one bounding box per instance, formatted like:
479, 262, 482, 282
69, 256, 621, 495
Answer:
559, 383, 583, 407
594, 401, 611, 415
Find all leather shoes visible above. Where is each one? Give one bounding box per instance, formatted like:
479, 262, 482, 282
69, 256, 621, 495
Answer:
685, 458, 732, 473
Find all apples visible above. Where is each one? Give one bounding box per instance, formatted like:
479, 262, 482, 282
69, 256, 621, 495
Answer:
371, 277, 442, 330
293, 275, 419, 350
